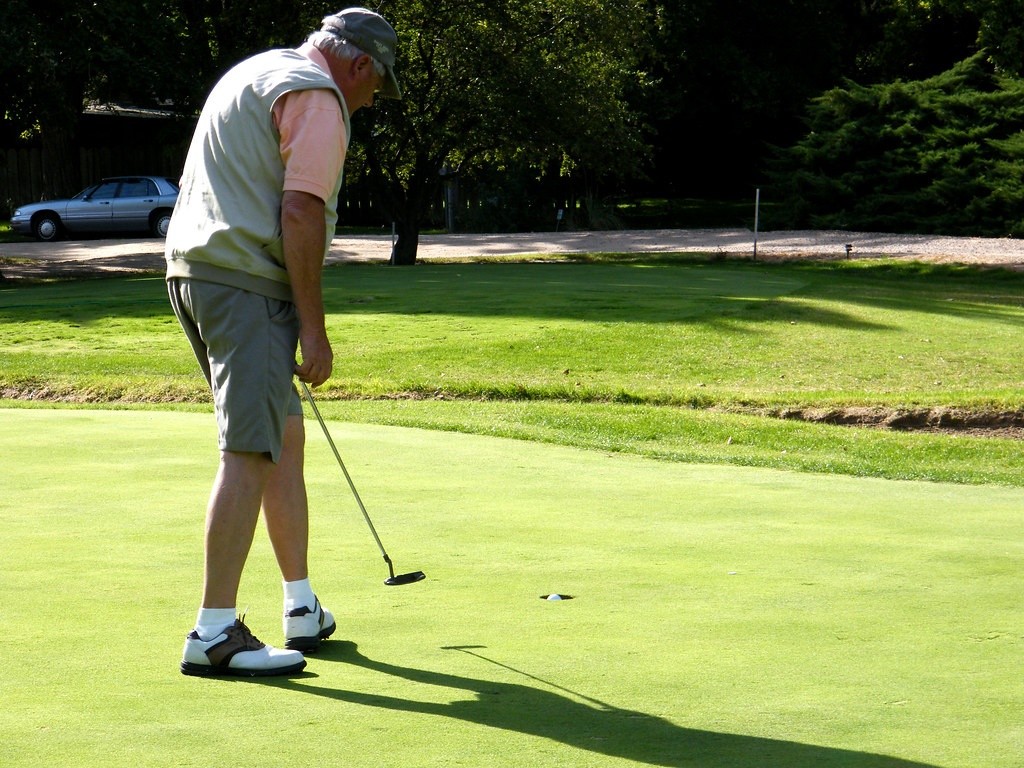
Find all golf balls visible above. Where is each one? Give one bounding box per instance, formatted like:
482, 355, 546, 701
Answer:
546, 594, 562, 601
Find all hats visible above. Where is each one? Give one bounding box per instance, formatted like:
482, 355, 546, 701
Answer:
321, 8, 401, 104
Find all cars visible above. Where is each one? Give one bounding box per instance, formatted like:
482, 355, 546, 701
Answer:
10, 175, 180, 242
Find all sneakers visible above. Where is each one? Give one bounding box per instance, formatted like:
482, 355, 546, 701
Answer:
181, 622, 306, 677
283, 595, 335, 652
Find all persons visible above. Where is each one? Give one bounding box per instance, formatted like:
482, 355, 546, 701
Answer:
165, 6, 403, 678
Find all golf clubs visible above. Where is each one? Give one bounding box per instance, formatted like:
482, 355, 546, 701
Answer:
294, 356, 427, 586
